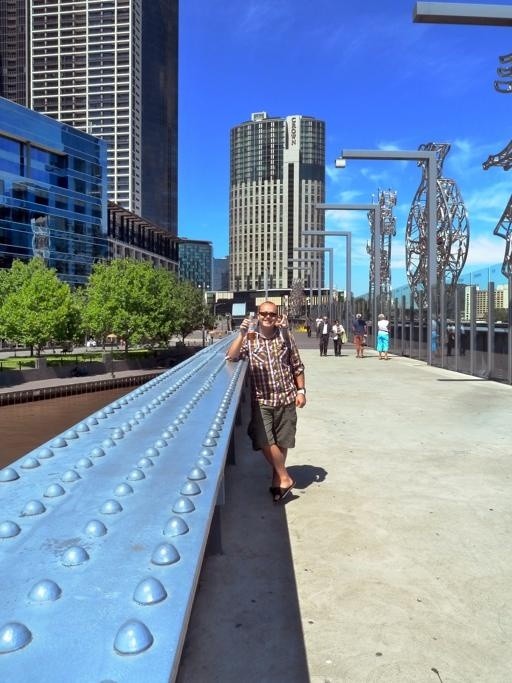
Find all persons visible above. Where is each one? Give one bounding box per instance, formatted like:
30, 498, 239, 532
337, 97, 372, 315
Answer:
377, 314, 390, 360
225, 301, 306, 503
352, 313, 367, 358
303, 316, 345, 356
431, 314, 465, 356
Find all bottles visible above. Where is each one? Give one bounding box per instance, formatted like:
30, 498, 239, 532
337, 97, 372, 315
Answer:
246, 311, 257, 341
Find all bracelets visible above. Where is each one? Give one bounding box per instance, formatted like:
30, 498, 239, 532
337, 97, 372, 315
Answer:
297, 387, 306, 394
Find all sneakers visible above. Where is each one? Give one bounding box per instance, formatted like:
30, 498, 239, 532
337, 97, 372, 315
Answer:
269, 475, 299, 502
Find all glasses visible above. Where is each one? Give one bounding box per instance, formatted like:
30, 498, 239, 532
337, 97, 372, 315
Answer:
259, 312, 277, 317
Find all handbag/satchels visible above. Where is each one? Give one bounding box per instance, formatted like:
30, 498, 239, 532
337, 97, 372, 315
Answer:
341, 333, 347, 343
